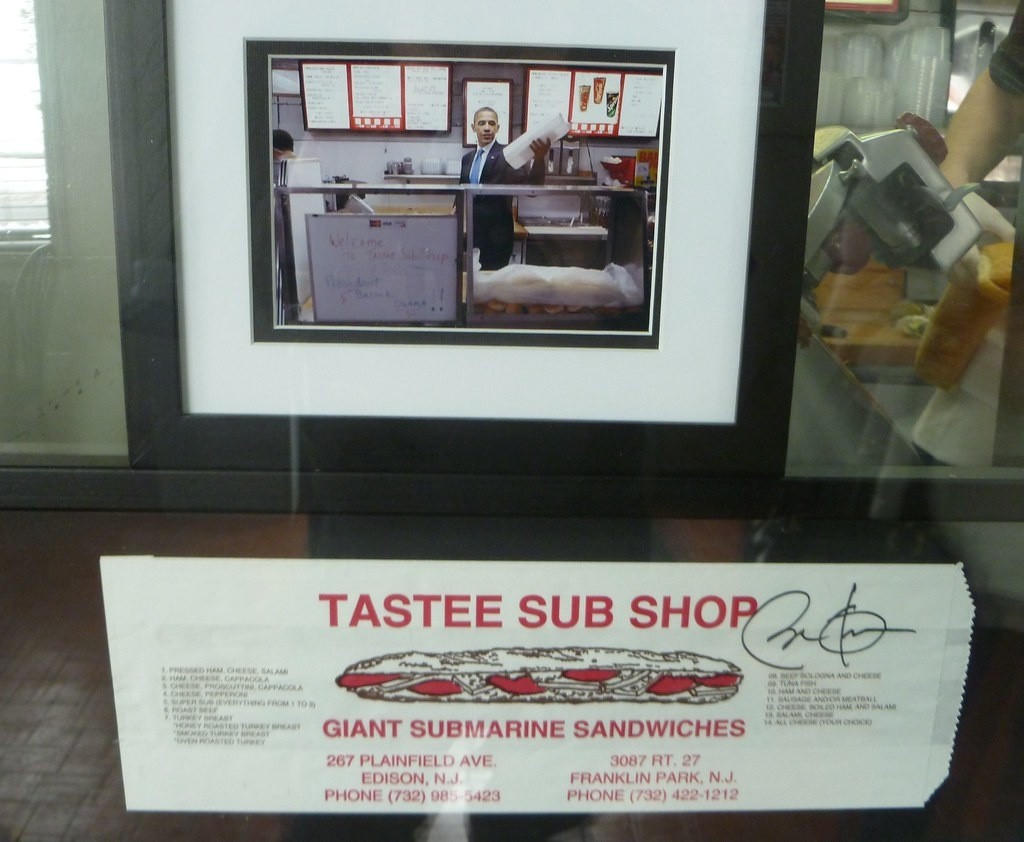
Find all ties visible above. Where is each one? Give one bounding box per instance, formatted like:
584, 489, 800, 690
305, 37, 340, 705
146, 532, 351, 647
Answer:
470, 149, 483, 183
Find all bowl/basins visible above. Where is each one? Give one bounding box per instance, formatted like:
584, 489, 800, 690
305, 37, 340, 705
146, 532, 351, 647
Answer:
420, 158, 462, 175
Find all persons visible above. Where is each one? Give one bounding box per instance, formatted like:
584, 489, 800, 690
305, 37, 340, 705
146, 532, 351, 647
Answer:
455, 107, 552, 271
916, 0, 1024, 294
274, 128, 294, 161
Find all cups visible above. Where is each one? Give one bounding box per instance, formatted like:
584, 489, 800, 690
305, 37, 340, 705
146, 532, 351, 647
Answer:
594, 78, 606, 104
607, 93, 619, 117
579, 85, 591, 111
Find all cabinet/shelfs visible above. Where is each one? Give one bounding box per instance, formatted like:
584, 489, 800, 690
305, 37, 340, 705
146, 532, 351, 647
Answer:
276, 182, 649, 330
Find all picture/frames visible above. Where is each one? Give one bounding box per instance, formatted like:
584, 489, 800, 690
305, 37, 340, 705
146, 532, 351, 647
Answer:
243, 37, 676, 351
